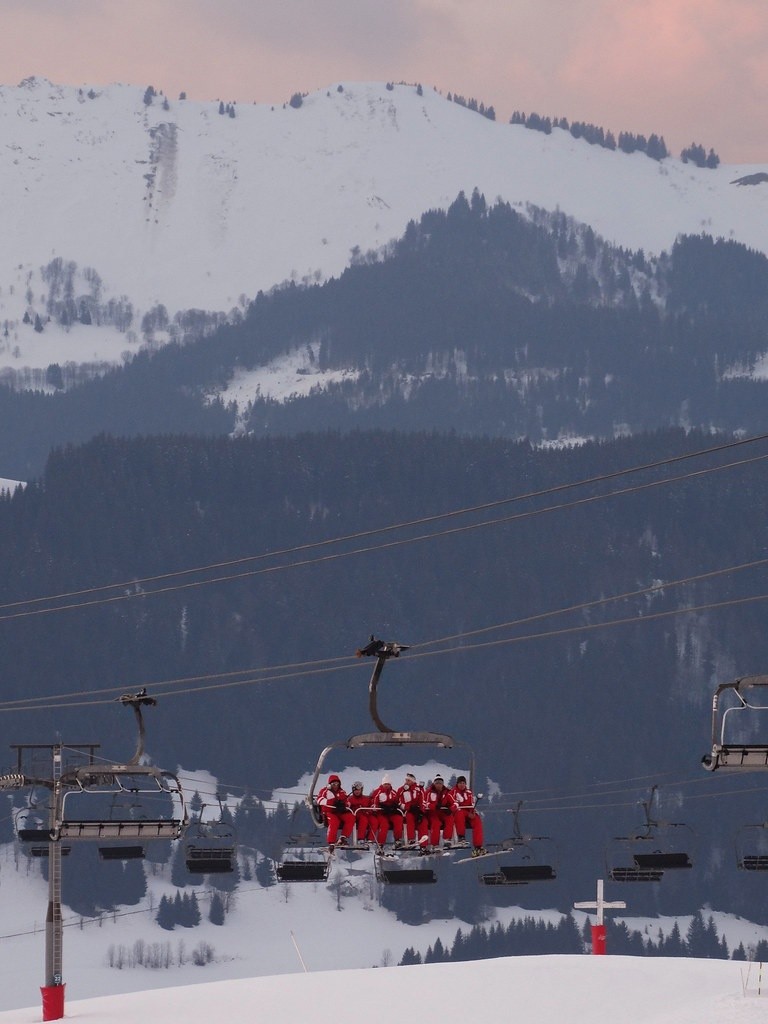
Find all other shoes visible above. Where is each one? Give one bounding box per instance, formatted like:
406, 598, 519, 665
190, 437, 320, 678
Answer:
431, 845, 440, 852
357, 840, 365, 847
443, 840, 452, 849
407, 840, 416, 850
470, 846, 489, 857
376, 843, 384, 855
458, 839, 471, 846
394, 841, 405, 849
420, 846, 429, 853
364, 840, 374, 847
328, 844, 335, 851
337, 836, 350, 847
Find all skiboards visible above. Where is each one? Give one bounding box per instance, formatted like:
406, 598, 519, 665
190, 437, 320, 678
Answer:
398, 835, 429, 849
453, 848, 513, 864
411, 853, 452, 861
319, 844, 371, 859
378, 852, 400, 861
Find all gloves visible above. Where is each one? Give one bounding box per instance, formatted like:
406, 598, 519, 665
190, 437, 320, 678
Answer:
326, 802, 472, 822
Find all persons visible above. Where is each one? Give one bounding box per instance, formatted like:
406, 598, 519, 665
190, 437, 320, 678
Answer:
370, 775, 403, 855
317, 775, 356, 853
397, 774, 431, 855
348, 781, 379, 846
424, 774, 455, 853
448, 776, 488, 857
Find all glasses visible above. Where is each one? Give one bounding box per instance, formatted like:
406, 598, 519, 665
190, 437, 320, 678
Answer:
352, 787, 360, 790
330, 783, 340, 788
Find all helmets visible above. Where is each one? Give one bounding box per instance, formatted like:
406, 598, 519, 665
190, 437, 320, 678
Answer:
352, 780, 364, 788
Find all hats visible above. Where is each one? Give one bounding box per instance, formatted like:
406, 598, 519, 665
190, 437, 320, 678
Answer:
381, 774, 391, 786
457, 776, 466, 784
328, 775, 340, 783
405, 773, 416, 784
434, 774, 444, 785
